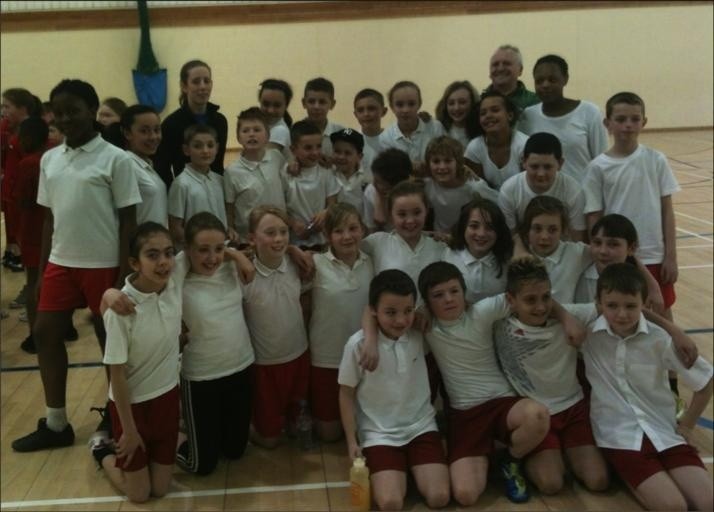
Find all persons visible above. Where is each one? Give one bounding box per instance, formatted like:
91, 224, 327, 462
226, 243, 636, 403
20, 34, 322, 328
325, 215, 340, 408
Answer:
99, 212, 316, 475
357, 261, 586, 507
334, 268, 450, 511
411, 259, 700, 495
569, 262, 714, 511
87, 221, 256, 503
0, 44, 679, 451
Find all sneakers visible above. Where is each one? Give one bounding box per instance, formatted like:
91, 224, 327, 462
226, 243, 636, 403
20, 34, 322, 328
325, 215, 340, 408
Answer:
672, 391, 685, 422
499, 450, 530, 503
2, 252, 24, 272
21, 327, 79, 352
11, 416, 75, 452
0, 285, 37, 323
88, 399, 112, 456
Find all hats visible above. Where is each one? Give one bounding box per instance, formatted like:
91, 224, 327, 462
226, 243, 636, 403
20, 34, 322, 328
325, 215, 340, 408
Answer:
331, 128, 364, 147
